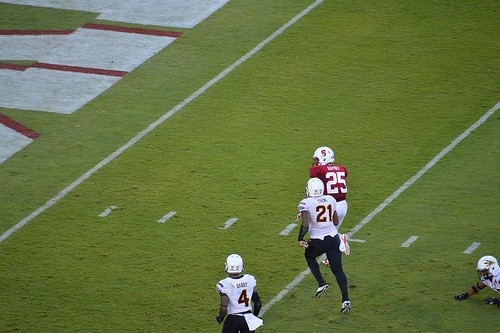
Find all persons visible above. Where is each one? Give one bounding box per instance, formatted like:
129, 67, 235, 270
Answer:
215, 254, 264, 333
310, 147, 350, 266
297, 177, 351, 315
457, 255, 500, 307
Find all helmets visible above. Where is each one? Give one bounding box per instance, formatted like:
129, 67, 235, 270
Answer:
477, 255, 498, 279
225, 253, 244, 274
312, 146, 335, 167
306, 177, 325, 198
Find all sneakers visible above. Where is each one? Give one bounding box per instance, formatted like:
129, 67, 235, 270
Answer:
315, 283, 329, 299
321, 257, 331, 266
340, 300, 351, 316
341, 233, 350, 256
